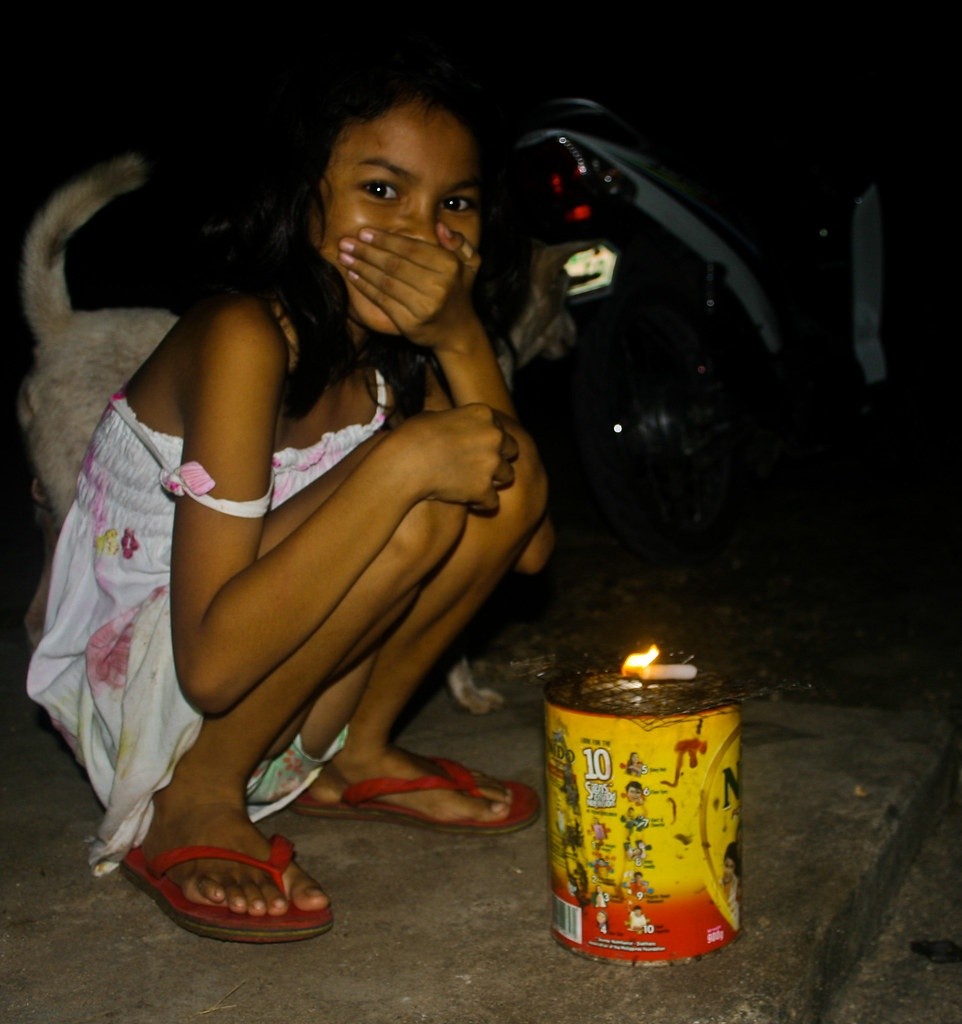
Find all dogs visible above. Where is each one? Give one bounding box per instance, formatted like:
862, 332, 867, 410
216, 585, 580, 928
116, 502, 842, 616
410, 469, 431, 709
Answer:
13, 153, 639, 714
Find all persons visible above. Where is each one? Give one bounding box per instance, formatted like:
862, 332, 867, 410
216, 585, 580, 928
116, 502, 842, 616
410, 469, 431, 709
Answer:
26, 75, 556, 944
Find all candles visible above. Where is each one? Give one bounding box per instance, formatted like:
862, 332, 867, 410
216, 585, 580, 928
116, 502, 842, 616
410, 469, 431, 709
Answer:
621, 645, 696, 680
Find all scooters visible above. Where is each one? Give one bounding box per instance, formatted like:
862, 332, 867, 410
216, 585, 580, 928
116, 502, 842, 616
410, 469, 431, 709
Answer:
496, 80, 920, 568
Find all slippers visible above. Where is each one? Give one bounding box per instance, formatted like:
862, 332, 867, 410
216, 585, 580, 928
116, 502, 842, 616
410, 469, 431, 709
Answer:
290, 755, 539, 833
121, 834, 334, 942
